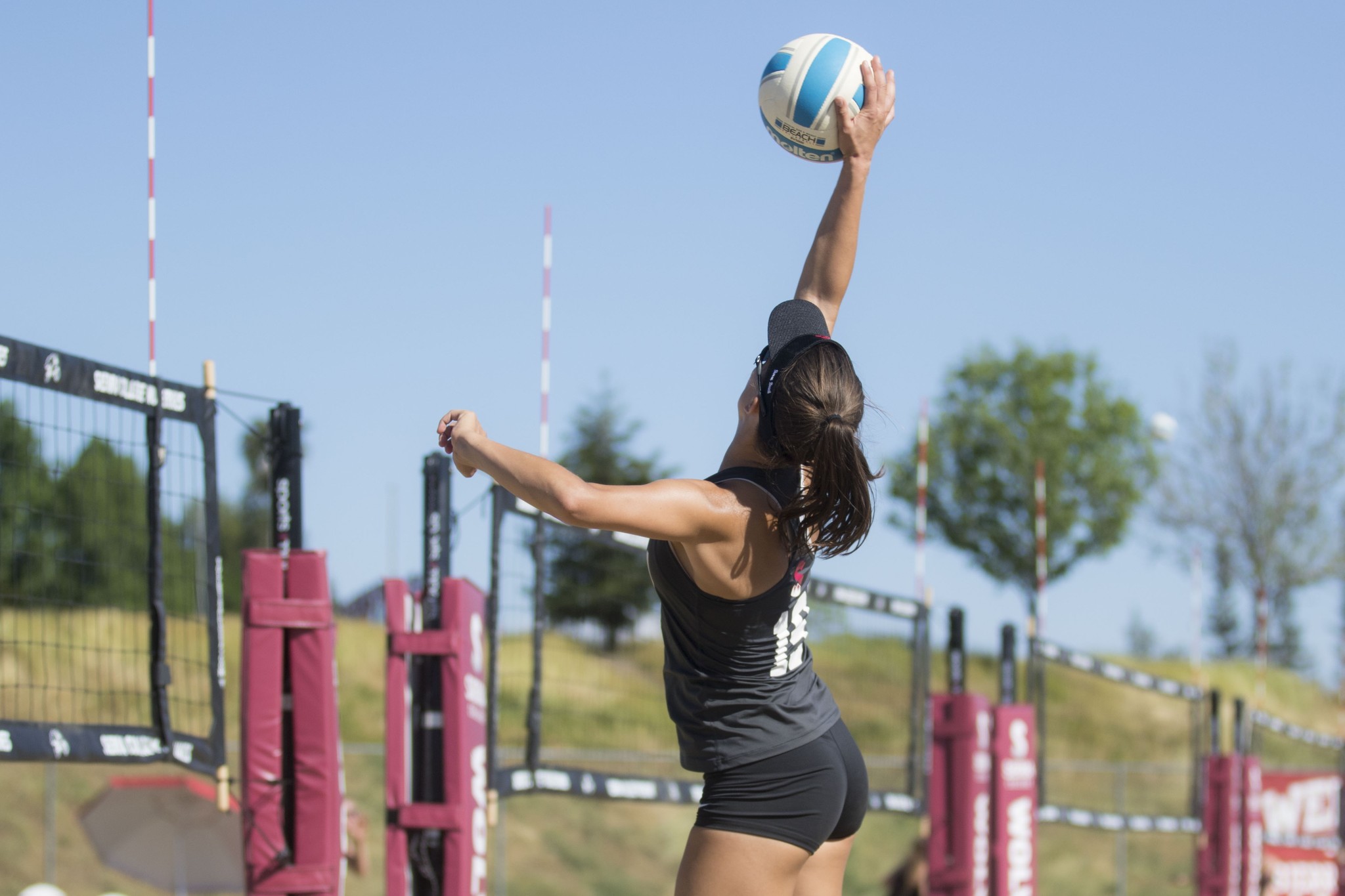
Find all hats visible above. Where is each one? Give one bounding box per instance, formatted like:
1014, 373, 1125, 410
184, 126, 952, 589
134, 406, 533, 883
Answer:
760, 299, 849, 403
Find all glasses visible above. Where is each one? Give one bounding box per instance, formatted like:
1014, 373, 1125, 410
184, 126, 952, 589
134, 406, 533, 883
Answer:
754, 345, 771, 416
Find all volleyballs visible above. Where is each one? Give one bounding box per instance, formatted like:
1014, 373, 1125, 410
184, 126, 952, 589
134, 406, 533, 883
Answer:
1148, 412, 1181, 444
757, 33, 875, 164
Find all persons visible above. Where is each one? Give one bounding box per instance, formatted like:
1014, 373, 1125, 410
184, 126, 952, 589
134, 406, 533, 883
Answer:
436, 53, 897, 896
886, 817, 934, 896
